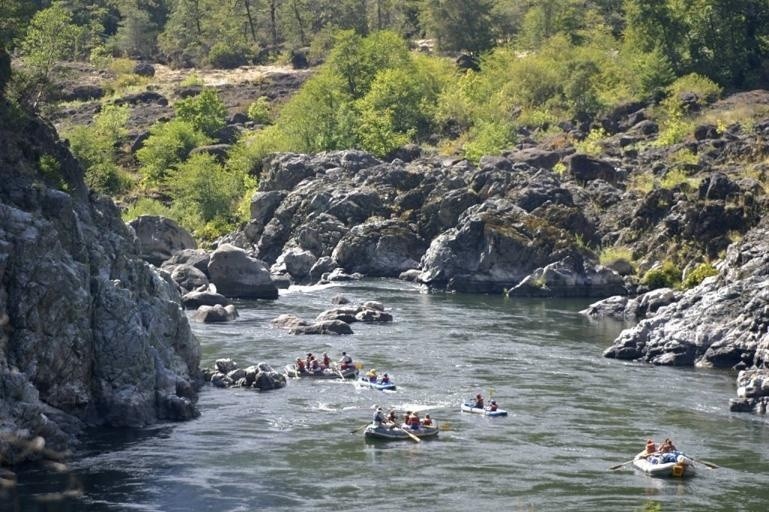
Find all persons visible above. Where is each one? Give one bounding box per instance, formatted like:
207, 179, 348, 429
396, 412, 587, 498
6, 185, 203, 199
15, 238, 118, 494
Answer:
659, 438, 671, 450
373, 407, 433, 429
645, 439, 655, 454
489, 399, 497, 412
473, 392, 484, 409
295, 351, 390, 385
662, 440, 675, 451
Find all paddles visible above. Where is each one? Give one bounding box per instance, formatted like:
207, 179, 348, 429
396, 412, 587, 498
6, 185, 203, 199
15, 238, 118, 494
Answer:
608, 454, 652, 470
389, 419, 421, 442
685, 455, 719, 469
350, 422, 373, 433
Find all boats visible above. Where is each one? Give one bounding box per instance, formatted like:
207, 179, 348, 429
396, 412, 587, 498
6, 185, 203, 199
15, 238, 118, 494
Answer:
364, 421, 438, 440
358, 376, 397, 392
631, 448, 697, 479
322, 361, 360, 381
460, 401, 508, 418
282, 359, 334, 379
